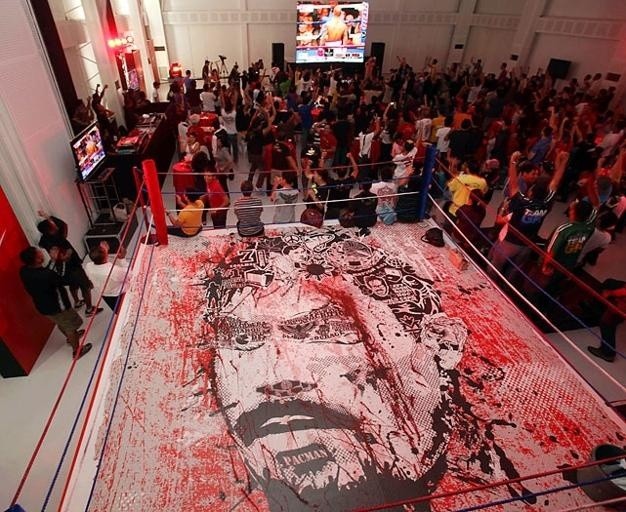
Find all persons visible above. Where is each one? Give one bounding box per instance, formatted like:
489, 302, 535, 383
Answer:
485, 180, 626, 360
296, 1, 362, 61
19, 209, 134, 359
70, 83, 116, 153
121, 55, 626, 237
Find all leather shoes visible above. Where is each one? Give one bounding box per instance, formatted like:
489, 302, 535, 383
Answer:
588, 345, 616, 362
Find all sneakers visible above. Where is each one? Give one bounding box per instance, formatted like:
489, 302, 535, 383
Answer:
73, 343, 92, 360
85, 306, 104, 318
66, 329, 85, 345
74, 299, 85, 310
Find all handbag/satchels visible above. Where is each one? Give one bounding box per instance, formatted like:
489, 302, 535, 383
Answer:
421, 228, 444, 247
300, 208, 323, 229
375, 201, 398, 225
229, 169, 234, 181
449, 248, 469, 271
339, 208, 354, 227
110, 203, 126, 221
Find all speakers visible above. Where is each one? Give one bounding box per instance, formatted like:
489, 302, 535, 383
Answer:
371, 42, 385, 72
546, 58, 572, 80
272, 43, 284, 72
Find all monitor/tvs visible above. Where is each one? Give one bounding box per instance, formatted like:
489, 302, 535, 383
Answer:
296, 0, 369, 64
69, 120, 108, 184
